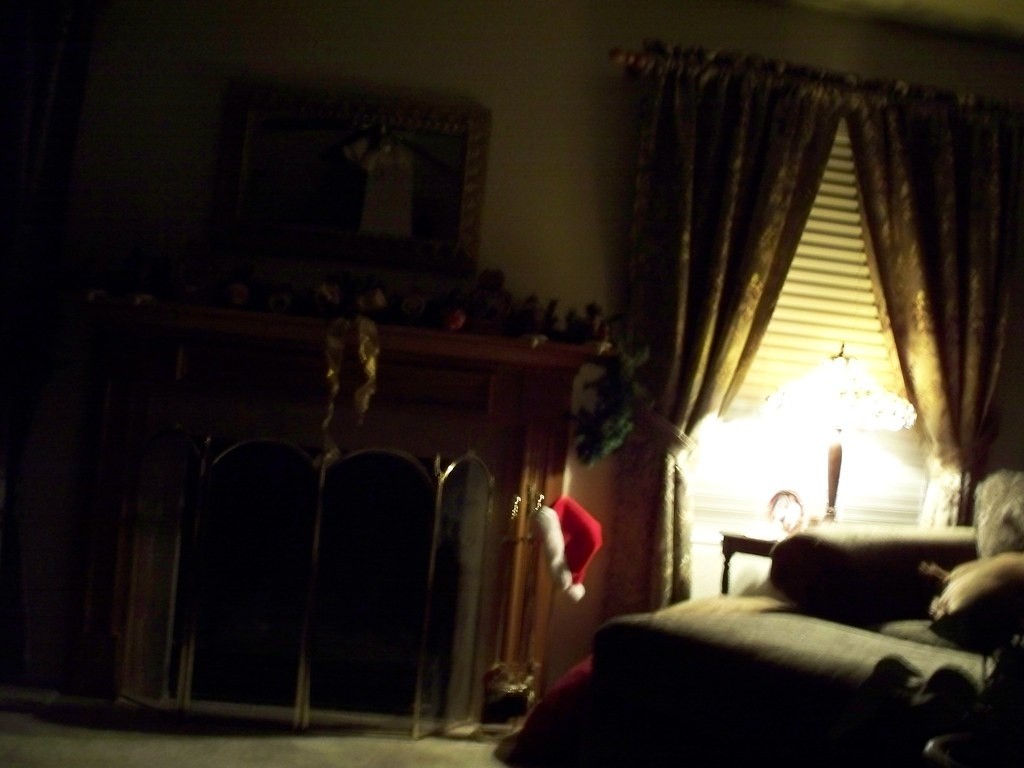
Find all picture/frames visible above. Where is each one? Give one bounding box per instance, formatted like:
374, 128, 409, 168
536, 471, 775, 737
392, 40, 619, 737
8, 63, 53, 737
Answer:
208, 80, 492, 272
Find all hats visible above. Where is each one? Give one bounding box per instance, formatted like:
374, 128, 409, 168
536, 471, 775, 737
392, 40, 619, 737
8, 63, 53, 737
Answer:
537, 496, 604, 603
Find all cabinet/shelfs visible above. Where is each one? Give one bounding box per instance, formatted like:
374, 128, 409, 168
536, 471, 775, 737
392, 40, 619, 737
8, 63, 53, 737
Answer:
39, 279, 611, 733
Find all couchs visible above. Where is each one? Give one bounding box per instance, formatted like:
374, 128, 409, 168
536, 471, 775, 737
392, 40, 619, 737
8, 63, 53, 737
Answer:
492, 526, 1024, 768
